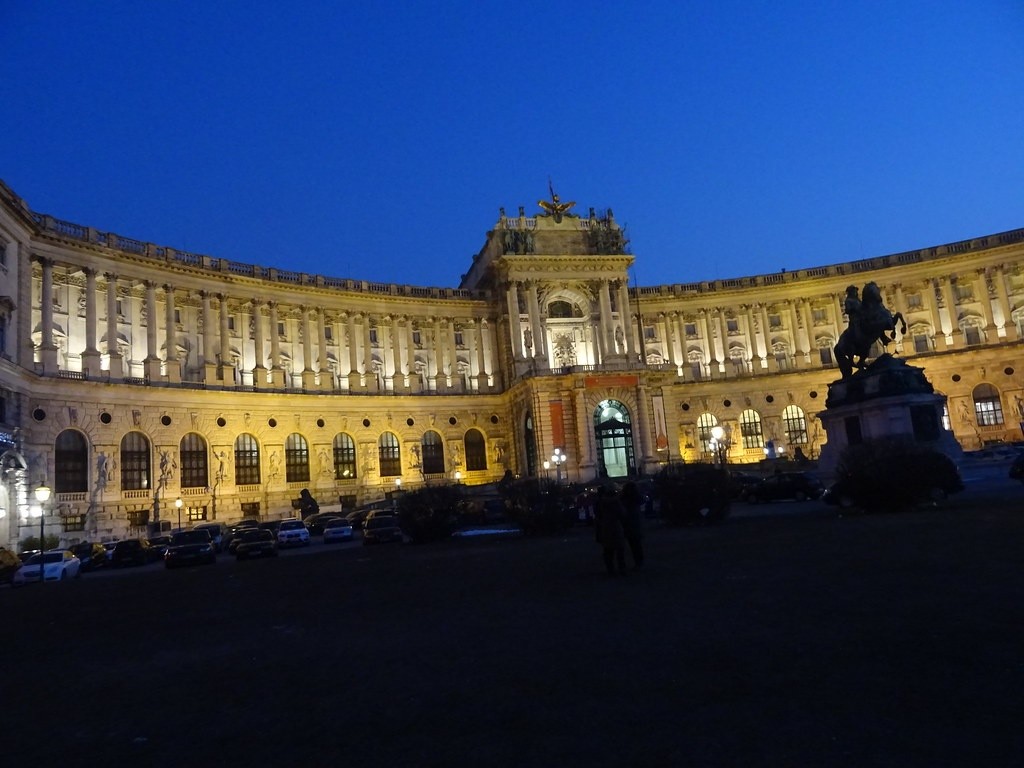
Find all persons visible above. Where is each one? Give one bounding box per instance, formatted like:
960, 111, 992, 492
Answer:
498, 471, 517, 497
594, 481, 646, 577
163, 450, 175, 478
524, 327, 532, 349
685, 394, 1024, 447
213, 438, 505, 477
844, 286, 890, 346
616, 324, 624, 346
102, 453, 116, 481
795, 447, 809, 466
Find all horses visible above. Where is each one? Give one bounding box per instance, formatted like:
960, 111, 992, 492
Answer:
834, 281, 907, 379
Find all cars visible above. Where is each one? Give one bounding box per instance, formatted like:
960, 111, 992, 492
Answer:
158, 507, 405, 570
741, 471, 826, 505
13, 550, 81, 588
0, 549, 23, 585
451, 479, 657, 527
16, 537, 150, 573
